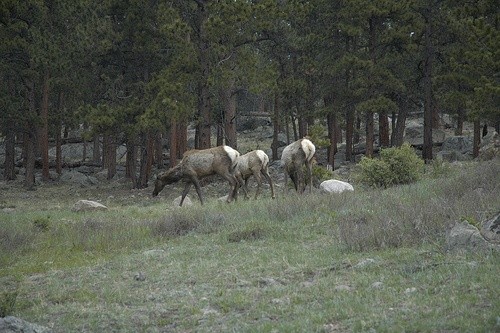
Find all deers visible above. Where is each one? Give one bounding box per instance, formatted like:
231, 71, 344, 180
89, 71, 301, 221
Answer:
280, 137, 316, 194
232, 149, 276, 201
151, 145, 250, 207
